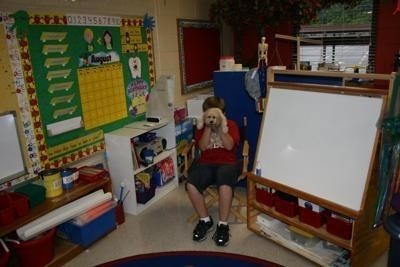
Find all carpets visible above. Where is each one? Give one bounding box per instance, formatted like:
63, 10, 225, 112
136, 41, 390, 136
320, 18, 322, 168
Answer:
96, 251, 284, 267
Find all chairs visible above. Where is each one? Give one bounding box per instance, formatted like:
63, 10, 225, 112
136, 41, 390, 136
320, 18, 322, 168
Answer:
182, 138, 249, 225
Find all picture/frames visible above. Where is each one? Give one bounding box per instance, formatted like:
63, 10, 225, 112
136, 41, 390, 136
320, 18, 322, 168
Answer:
177, 18, 223, 96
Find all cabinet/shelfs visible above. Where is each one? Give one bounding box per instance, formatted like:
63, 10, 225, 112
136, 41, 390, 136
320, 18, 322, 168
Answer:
104, 120, 179, 216
1, 165, 116, 266
247, 66, 400, 267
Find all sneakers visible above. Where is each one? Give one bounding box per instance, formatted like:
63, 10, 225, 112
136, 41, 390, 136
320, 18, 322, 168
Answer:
191, 215, 214, 242
213, 223, 231, 246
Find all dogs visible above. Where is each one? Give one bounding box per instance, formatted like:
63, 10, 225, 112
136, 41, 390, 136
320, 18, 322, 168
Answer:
197, 107, 228, 149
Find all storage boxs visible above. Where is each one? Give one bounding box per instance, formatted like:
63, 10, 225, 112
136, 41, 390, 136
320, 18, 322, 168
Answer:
255, 188, 275, 208
288, 226, 319, 249
326, 216, 352, 240
14, 182, 46, 206
60, 202, 118, 247
298, 206, 328, 227
275, 197, 297, 218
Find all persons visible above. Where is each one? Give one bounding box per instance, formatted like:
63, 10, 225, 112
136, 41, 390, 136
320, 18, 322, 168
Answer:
185, 97, 241, 247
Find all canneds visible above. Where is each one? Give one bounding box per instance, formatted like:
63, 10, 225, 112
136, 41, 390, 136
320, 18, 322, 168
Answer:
39, 169, 62, 197
63, 168, 74, 189
73, 167, 79, 183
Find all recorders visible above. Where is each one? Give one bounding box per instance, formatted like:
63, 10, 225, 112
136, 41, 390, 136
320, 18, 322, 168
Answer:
136, 137, 167, 163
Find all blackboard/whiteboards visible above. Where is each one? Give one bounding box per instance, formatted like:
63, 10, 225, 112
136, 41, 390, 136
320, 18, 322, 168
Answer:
253, 84, 387, 218
0, 110, 27, 185
